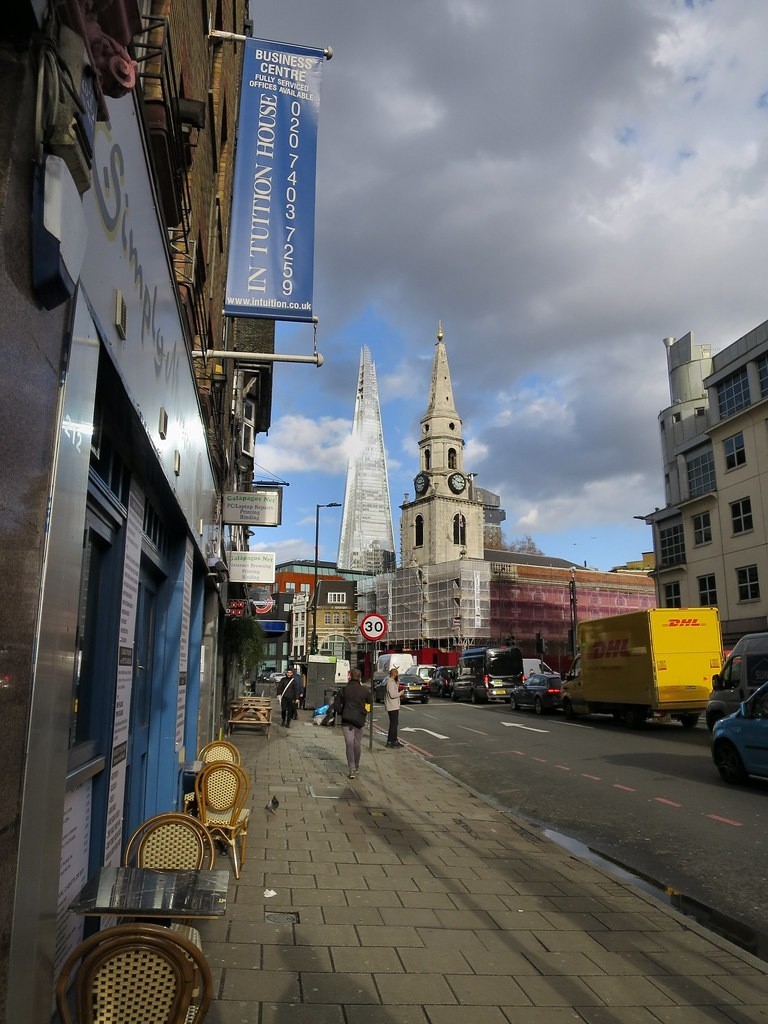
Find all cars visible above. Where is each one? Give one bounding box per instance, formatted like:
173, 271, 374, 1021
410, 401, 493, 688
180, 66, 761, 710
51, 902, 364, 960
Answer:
270, 673, 286, 682
711, 682, 768, 784
373, 674, 430, 704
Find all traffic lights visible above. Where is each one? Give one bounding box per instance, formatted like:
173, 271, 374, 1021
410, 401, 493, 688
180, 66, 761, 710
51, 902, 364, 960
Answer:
507, 636, 515, 647
540, 638, 548, 653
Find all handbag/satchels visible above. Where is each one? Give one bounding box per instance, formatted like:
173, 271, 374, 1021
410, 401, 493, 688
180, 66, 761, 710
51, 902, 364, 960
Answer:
364, 703, 371, 712
276, 694, 282, 711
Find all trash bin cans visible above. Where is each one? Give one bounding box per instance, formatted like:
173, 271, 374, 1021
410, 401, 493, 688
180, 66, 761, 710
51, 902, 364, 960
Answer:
324, 687, 343, 726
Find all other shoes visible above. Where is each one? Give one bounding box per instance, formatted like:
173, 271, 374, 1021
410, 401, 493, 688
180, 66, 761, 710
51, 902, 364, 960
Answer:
349, 773, 356, 779
386, 741, 393, 748
286, 725, 290, 728
355, 768, 359, 774
391, 742, 404, 749
281, 721, 285, 727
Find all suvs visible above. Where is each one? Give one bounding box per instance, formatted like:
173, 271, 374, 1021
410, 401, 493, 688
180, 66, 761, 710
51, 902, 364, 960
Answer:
510, 673, 565, 715
429, 666, 456, 697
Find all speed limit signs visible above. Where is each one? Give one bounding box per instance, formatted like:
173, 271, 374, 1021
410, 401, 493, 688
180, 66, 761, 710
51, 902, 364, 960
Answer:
360, 613, 387, 641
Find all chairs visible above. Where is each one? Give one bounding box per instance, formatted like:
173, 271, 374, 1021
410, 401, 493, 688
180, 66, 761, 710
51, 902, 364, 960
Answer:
196, 760, 250, 880
57, 924, 214, 1024
116, 811, 214, 1024
184, 741, 241, 855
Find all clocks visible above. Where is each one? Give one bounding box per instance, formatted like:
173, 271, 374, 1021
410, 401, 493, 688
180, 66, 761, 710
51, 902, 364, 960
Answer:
415, 474, 428, 494
448, 472, 467, 494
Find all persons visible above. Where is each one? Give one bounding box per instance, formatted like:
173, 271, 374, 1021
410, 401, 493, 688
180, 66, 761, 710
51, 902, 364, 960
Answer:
277, 668, 299, 728
383, 669, 405, 748
291, 669, 303, 720
529, 669, 536, 676
333, 669, 372, 779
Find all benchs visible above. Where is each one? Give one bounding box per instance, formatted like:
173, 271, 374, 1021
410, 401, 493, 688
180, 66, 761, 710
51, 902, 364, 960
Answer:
228, 720, 272, 724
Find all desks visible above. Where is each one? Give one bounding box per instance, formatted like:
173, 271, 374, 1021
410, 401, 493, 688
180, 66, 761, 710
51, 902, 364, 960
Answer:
184, 762, 240, 856
228, 696, 273, 740
67, 866, 230, 928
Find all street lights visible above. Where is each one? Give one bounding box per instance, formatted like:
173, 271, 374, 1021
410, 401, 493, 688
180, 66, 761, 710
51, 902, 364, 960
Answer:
310, 502, 342, 657
494, 567, 505, 647
568, 566, 577, 659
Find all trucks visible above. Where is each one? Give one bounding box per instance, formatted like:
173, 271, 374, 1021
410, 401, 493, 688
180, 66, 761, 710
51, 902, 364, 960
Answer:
560, 607, 725, 729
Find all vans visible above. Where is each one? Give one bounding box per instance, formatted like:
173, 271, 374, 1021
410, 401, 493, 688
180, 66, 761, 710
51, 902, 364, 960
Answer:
405, 665, 436, 684
705, 633, 768, 730
377, 653, 414, 675
450, 646, 525, 704
523, 659, 560, 680
335, 660, 351, 688
